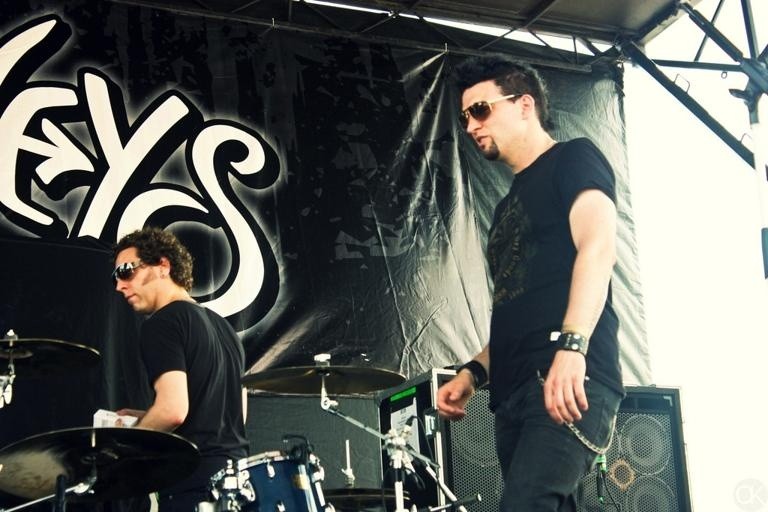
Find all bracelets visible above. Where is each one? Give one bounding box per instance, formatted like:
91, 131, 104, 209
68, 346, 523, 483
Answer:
456, 360, 488, 392
556, 333, 590, 357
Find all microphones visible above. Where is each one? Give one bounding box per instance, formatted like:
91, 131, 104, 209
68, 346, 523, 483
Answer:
595, 461, 605, 503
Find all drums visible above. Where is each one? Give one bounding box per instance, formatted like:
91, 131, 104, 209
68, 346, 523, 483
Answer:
195, 448, 336, 512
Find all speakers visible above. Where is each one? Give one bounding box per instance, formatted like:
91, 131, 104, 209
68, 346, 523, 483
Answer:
376, 367, 504, 512
572, 386, 695, 511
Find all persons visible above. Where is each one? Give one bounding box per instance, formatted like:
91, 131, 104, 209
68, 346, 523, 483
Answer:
113, 229, 249, 498
437, 54, 625, 512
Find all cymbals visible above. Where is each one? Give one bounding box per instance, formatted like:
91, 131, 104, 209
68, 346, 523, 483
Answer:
0, 339, 100, 371
1, 427, 204, 503
241, 366, 406, 395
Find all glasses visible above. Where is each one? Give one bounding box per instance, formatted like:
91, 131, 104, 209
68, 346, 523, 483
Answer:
459, 93, 523, 130
112, 258, 144, 288
